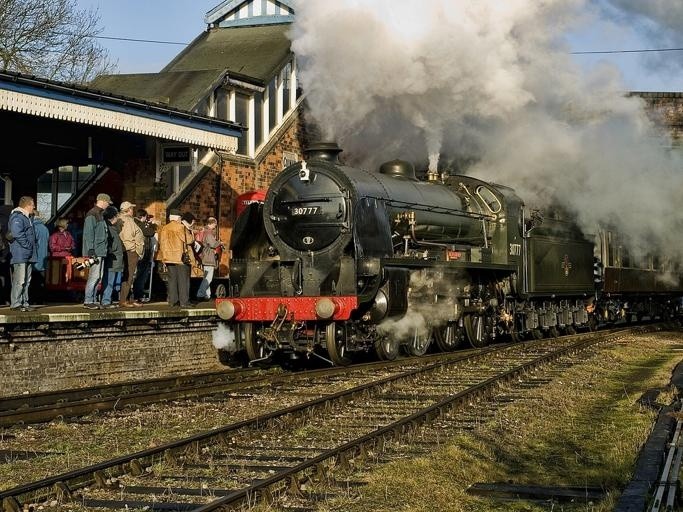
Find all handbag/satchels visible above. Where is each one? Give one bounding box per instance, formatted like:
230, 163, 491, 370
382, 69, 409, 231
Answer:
181, 252, 190, 264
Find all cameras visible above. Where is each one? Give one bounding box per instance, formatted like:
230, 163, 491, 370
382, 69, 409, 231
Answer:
76, 255, 99, 271
146, 215, 154, 221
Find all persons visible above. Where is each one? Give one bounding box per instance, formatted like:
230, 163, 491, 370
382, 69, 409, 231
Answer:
29, 209, 50, 305
5, 196, 39, 311
49, 217, 73, 257
82, 192, 221, 310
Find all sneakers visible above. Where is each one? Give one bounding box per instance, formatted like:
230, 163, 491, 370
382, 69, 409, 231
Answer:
84, 301, 143, 309
181, 304, 196, 308
11, 307, 35, 312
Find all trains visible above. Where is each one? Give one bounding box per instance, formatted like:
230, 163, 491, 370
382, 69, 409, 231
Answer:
209, 143, 681, 367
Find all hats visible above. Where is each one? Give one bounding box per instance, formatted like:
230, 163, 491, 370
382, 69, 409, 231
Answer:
170, 209, 183, 215
96, 193, 113, 204
58, 220, 67, 229
120, 202, 136, 210
